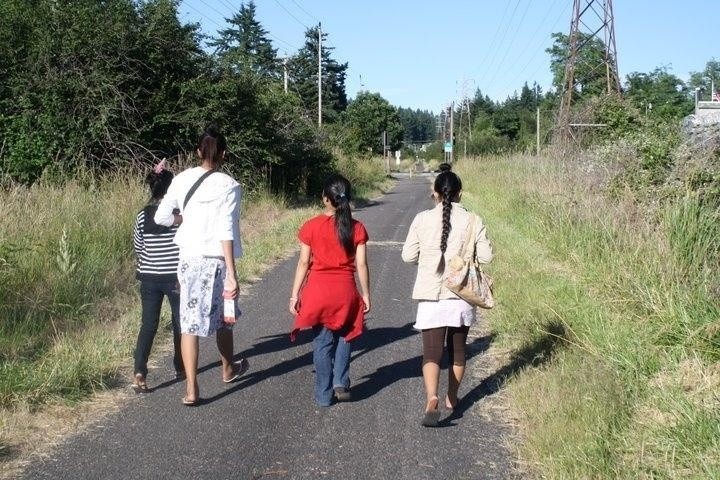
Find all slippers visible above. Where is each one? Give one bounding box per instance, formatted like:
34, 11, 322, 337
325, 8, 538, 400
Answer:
182, 396, 194, 405
132, 376, 151, 393
422, 409, 441, 427
223, 359, 250, 383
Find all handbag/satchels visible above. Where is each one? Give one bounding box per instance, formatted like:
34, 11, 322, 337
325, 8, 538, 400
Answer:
444, 255, 496, 309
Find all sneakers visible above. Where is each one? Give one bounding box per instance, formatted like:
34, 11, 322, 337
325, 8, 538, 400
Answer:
335, 386, 351, 401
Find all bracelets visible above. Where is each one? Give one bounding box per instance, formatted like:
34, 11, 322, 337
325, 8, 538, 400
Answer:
289, 296, 300, 303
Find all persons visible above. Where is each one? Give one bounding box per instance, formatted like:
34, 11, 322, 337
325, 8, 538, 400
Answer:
151, 129, 249, 408
400, 163, 495, 429
131, 157, 186, 393
289, 172, 372, 410
